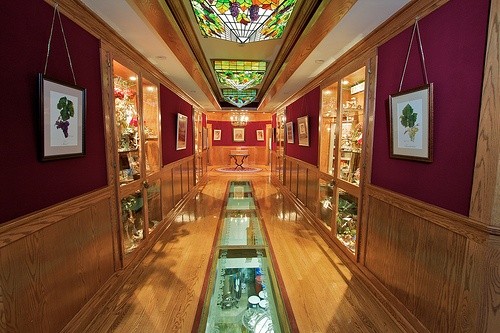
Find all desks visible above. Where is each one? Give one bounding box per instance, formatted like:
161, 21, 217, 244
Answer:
228, 154, 250, 170
242, 308, 268, 332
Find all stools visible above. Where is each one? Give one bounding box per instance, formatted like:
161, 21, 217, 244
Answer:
248, 291, 266, 309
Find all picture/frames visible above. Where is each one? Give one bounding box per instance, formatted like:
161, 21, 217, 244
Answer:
272, 128, 275, 142
286, 122, 294, 143
297, 114, 310, 147
233, 128, 245, 142
38, 74, 87, 161
256, 129, 265, 141
214, 130, 221, 140
388, 83, 435, 163
176, 113, 188, 150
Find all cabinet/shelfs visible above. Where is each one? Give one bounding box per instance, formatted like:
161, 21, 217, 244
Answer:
332, 147, 361, 172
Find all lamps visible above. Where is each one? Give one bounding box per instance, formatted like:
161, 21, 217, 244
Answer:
230, 112, 249, 126
